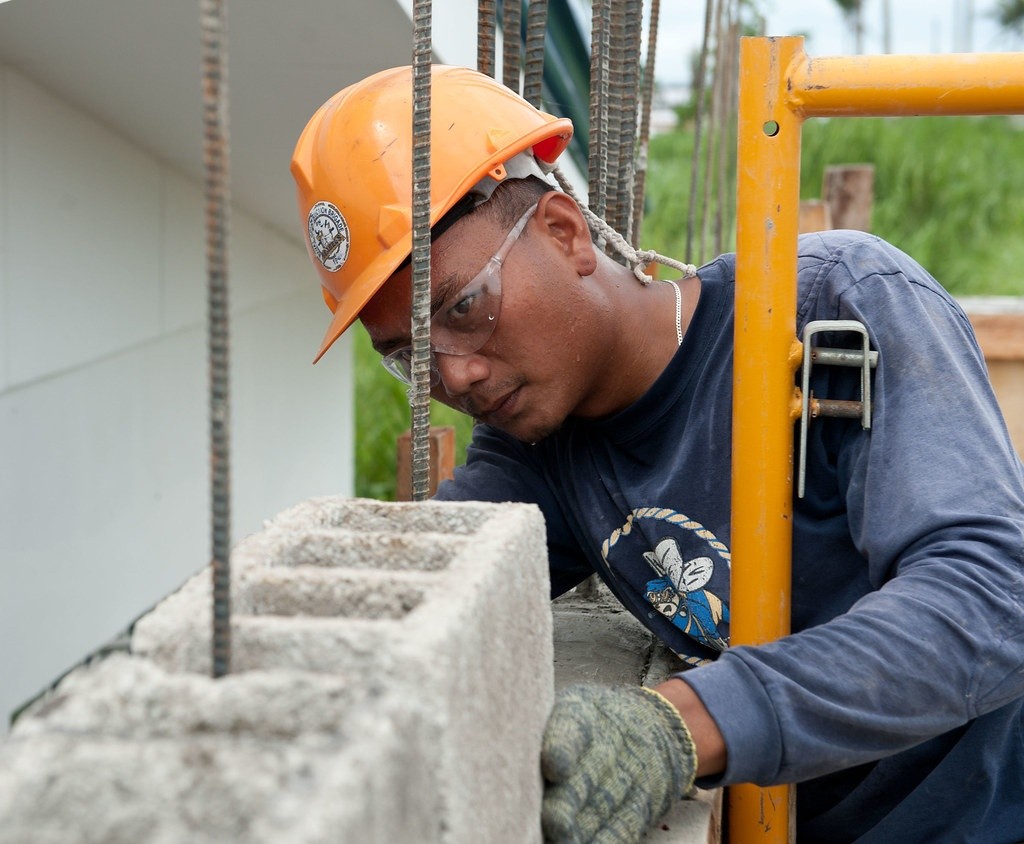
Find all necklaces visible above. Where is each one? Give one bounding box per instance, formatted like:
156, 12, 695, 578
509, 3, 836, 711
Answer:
661, 279, 683, 345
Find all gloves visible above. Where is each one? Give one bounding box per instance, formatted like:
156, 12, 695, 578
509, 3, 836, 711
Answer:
541, 681, 699, 844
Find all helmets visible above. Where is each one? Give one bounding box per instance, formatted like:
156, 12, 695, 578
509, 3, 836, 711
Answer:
288, 63, 573, 365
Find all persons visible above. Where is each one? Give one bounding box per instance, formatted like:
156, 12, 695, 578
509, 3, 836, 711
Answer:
290, 62, 1024, 844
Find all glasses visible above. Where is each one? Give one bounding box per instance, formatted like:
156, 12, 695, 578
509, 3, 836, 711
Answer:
379, 202, 541, 390
